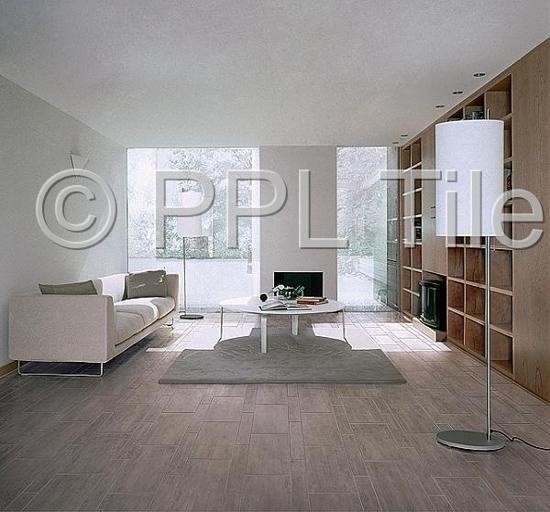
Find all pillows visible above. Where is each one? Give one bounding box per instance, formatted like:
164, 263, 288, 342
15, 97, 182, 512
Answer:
125, 269, 169, 298
36, 280, 99, 295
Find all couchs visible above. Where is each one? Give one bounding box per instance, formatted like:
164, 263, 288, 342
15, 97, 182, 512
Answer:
8, 270, 182, 378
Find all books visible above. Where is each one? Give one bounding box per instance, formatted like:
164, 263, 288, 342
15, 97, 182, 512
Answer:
258, 297, 328, 311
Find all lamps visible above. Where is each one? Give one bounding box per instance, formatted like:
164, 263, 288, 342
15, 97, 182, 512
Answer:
435, 117, 506, 456
179, 191, 203, 320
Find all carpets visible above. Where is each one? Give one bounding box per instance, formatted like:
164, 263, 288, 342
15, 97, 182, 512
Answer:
158, 346, 408, 384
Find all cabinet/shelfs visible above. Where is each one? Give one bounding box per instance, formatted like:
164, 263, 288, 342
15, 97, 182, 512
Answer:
395, 39, 550, 404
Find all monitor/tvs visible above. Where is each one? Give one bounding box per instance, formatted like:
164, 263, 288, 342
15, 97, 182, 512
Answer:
417, 279, 446, 330
